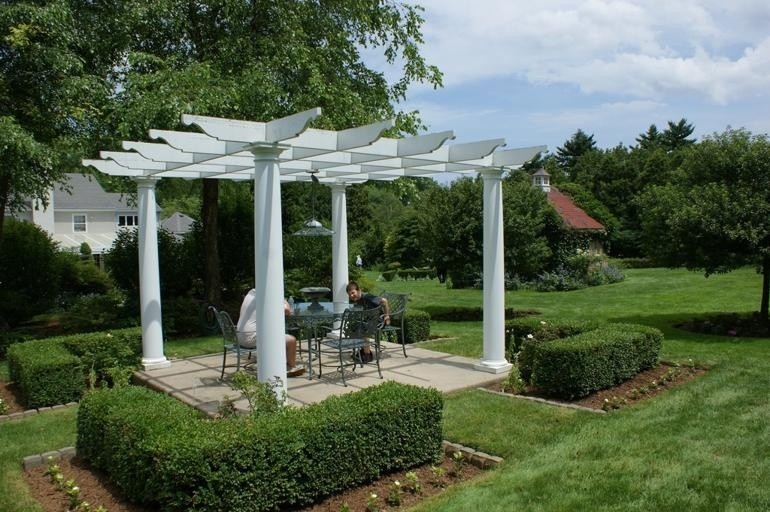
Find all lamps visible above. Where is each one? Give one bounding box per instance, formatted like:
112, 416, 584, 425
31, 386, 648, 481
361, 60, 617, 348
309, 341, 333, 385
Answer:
291, 169, 337, 239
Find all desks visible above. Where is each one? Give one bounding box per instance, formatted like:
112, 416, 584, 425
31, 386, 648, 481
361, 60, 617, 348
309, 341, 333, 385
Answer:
283, 301, 377, 378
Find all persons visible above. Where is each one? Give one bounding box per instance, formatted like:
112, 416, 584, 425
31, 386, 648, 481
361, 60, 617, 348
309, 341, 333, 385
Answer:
236, 288, 305, 377
346, 281, 391, 363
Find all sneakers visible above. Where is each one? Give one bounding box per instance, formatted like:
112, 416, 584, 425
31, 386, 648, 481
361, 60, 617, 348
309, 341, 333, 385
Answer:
355, 349, 364, 360
360, 351, 373, 364
287, 365, 305, 377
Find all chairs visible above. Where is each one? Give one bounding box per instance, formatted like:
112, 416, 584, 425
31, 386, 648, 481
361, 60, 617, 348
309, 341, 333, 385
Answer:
293, 284, 334, 363
315, 306, 386, 387
365, 289, 409, 362
214, 308, 256, 386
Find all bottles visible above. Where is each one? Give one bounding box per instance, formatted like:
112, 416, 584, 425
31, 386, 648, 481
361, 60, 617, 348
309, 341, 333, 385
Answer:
287, 296, 294, 314
